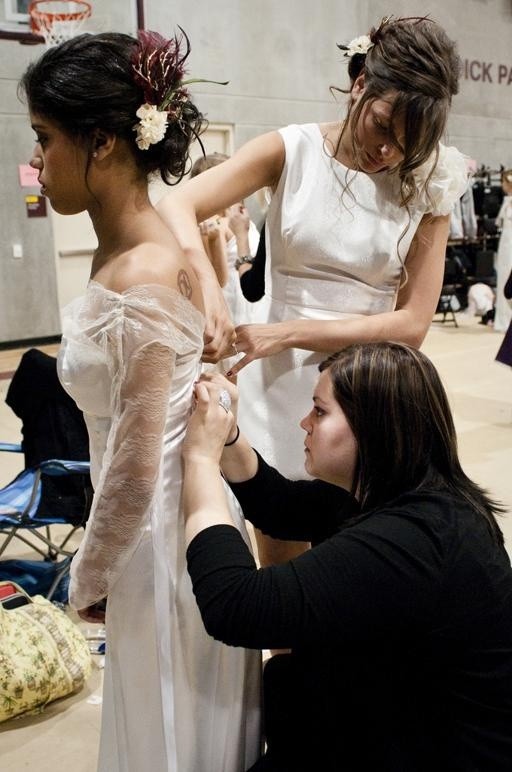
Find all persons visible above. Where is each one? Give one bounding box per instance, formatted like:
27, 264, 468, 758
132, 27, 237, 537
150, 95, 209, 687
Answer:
195, 150, 268, 327
495, 269, 512, 367
231, 203, 266, 302
189, 340, 507, 772
154, 12, 468, 657
17, 24, 261, 771
492, 170, 512, 331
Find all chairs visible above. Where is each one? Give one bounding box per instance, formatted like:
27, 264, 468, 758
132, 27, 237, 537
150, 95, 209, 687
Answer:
1, 348, 91, 603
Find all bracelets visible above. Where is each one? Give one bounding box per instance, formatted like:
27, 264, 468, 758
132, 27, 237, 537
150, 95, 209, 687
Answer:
224, 425, 240, 446
234, 256, 252, 269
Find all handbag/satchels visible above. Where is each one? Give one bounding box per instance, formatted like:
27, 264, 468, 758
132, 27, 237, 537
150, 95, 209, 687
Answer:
0, 580, 92, 723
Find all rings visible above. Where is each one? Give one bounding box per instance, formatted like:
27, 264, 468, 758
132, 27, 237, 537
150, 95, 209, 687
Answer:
220, 391, 232, 412
235, 346, 239, 354
